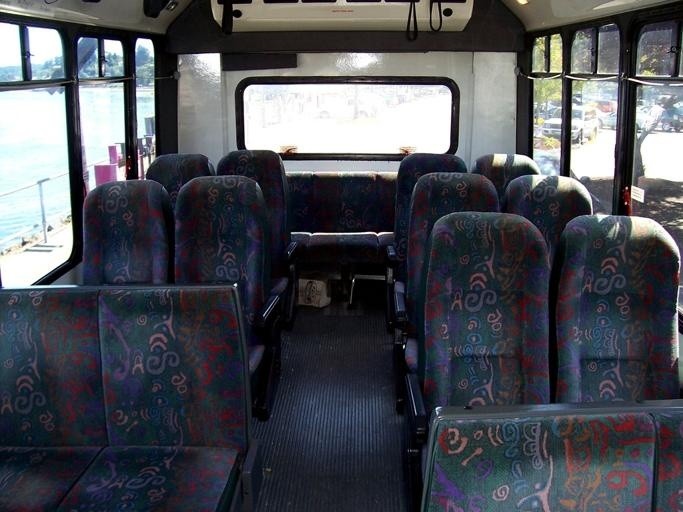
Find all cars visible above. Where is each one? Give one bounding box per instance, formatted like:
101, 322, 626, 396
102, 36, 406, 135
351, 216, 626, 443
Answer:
534, 150, 606, 214
533, 94, 683, 133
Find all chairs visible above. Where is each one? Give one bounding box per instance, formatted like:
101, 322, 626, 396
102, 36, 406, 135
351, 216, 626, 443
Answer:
386, 152, 540, 332
393, 171, 595, 364
2, 283, 265, 512
286, 172, 398, 312
145, 150, 299, 326
402, 398, 682, 511
84, 176, 283, 419
399, 209, 682, 442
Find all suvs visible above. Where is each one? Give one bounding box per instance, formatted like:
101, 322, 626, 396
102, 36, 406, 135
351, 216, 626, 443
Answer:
542, 106, 599, 145
